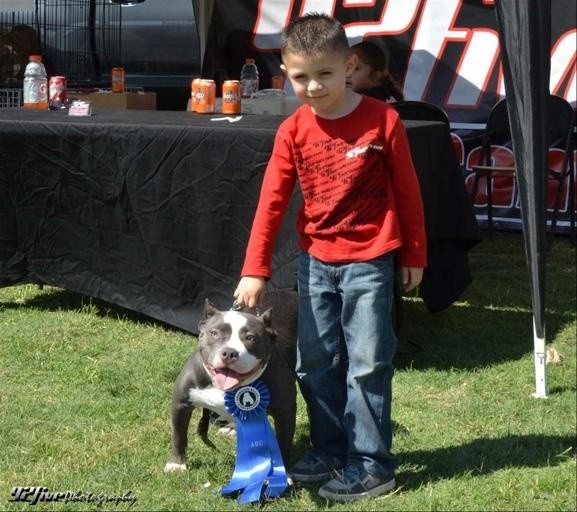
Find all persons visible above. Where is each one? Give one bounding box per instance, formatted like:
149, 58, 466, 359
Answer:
345, 40, 403, 107
233, 12, 429, 504
2, 24, 65, 89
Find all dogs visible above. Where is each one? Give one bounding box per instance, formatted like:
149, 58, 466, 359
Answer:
163, 297, 298, 485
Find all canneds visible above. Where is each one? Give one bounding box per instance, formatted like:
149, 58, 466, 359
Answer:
272, 75, 284, 89
191, 79, 201, 112
112, 68, 125, 92
223, 80, 241, 114
197, 80, 216, 113
49, 76, 67, 109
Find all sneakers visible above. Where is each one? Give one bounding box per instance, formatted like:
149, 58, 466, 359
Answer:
318, 465, 397, 500
291, 453, 343, 481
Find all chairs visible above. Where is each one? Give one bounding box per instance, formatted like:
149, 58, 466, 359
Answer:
382, 101, 447, 125
466, 95, 577, 250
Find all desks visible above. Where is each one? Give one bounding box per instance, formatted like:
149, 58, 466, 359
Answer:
0, 109, 480, 339
65, 91, 158, 111
186, 93, 300, 115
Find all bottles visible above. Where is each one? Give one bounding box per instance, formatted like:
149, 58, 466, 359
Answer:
240, 59, 259, 98
23, 55, 48, 109
